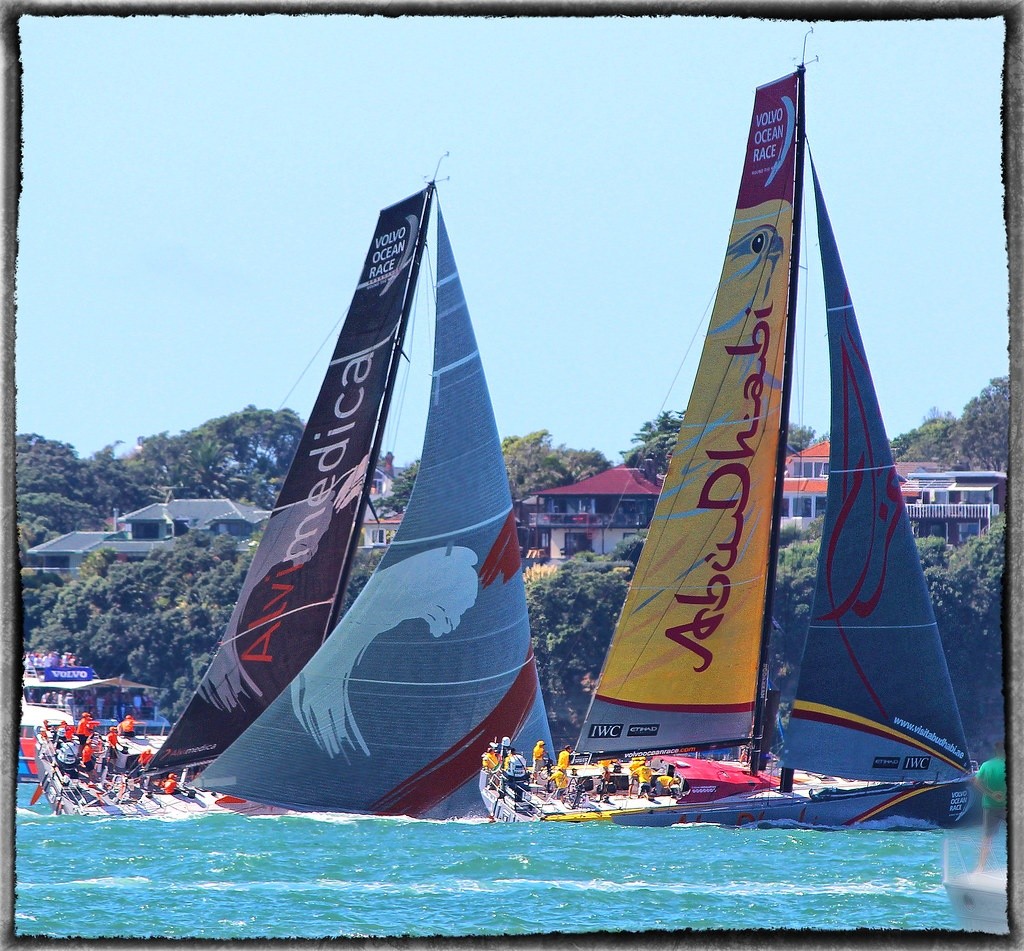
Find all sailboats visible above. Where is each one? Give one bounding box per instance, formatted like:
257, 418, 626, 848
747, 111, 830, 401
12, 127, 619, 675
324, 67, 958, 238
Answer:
479, 30, 985, 832
33, 150, 557, 819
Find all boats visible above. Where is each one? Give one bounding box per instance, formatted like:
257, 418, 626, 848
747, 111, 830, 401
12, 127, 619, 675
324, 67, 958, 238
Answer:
17, 663, 171, 786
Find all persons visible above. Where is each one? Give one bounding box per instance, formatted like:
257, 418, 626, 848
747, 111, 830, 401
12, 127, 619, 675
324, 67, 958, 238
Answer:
152, 777, 164, 787
83, 740, 96, 769
558, 745, 571, 771
41, 690, 74, 708
57, 720, 72, 739
974, 740, 1006, 872
75, 688, 159, 720
613, 758, 622, 773
107, 726, 123, 759
76, 712, 91, 755
629, 760, 680, 800
533, 740, 546, 771
481, 737, 527, 790
117, 715, 146, 736
572, 768, 578, 776
550, 766, 568, 800
22, 652, 83, 667
43, 719, 49, 731
164, 774, 180, 795
138, 749, 154, 767
27, 689, 34, 703
598, 766, 611, 792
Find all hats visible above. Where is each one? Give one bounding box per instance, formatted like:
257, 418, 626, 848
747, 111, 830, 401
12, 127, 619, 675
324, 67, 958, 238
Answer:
556, 766, 561, 770
60, 720, 66, 724
110, 727, 116, 731
638, 760, 645, 765
126, 715, 132, 719
564, 744, 571, 749
501, 737, 511, 747
44, 720, 49, 724
82, 712, 90, 716
537, 741, 545, 745
86, 739, 92, 744
675, 777, 681, 784
146, 749, 151, 754
168, 774, 177, 778
